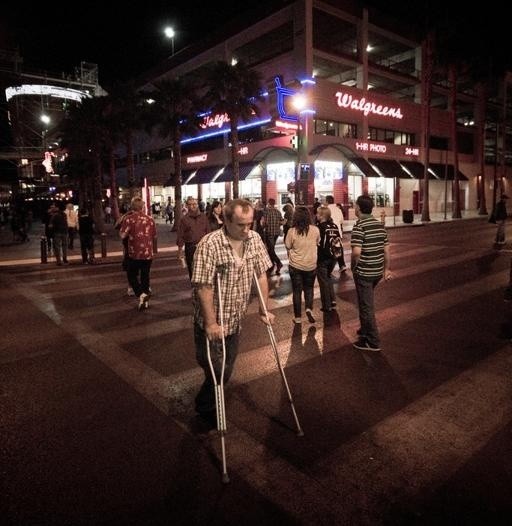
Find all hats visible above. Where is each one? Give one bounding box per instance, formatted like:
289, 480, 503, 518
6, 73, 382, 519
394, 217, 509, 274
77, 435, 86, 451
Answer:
499, 194, 510, 199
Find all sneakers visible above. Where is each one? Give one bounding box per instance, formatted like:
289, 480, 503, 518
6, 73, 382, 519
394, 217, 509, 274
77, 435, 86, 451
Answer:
275, 263, 283, 276
354, 339, 382, 353
338, 265, 347, 274
305, 308, 316, 324
81, 260, 89, 265
194, 396, 216, 430
127, 287, 135, 296
495, 241, 506, 245
89, 259, 95, 263
63, 260, 69, 264
56, 261, 62, 267
331, 301, 337, 310
149, 287, 153, 295
144, 300, 149, 309
137, 292, 149, 312
291, 316, 302, 324
320, 307, 332, 312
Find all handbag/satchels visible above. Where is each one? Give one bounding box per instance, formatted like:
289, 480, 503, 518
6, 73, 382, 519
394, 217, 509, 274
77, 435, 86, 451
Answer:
487, 214, 496, 224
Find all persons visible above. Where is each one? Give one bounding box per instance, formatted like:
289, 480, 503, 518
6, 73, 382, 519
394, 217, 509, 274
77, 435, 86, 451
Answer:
0, 196, 157, 311
493, 195, 509, 245
377, 195, 384, 207
503, 257, 512, 303
351, 195, 394, 352
152, 196, 347, 281
285, 208, 322, 324
191, 199, 275, 415
315, 207, 340, 312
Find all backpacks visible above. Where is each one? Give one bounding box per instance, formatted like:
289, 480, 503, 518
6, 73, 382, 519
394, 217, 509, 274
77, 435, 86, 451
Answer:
323, 225, 343, 260
